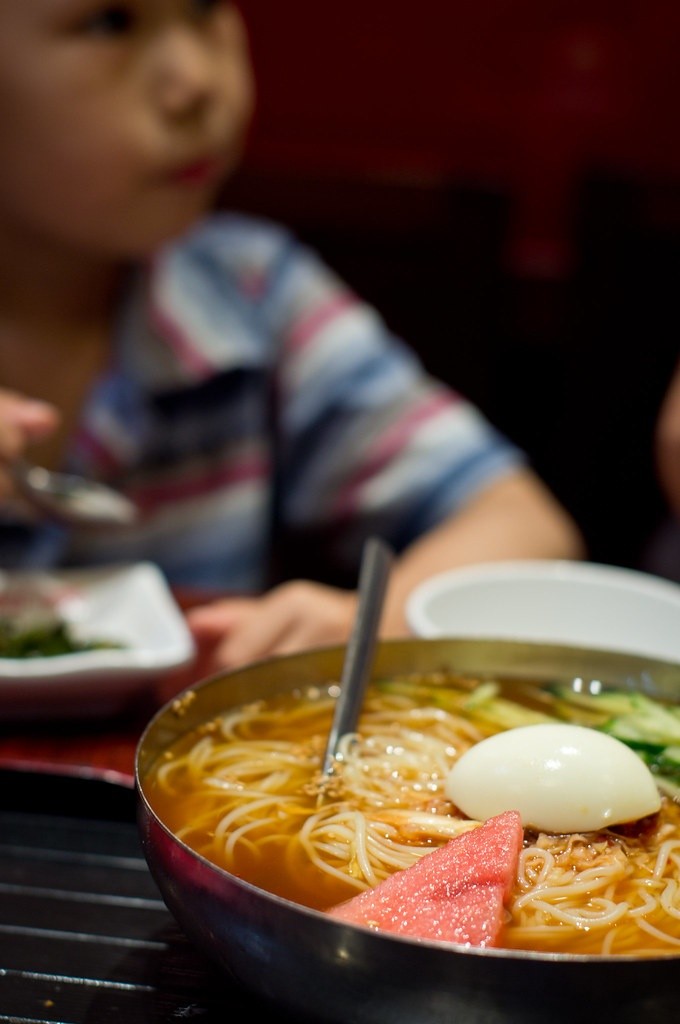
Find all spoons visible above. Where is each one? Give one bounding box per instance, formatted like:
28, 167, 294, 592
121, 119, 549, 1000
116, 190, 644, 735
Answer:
304, 533, 393, 820
0, 451, 142, 532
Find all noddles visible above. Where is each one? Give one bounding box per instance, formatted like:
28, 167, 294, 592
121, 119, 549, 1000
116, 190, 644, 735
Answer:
148, 676, 679, 959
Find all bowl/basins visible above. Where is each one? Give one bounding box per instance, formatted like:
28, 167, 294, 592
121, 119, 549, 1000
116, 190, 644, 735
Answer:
408, 557, 679, 669
134, 635, 678, 1022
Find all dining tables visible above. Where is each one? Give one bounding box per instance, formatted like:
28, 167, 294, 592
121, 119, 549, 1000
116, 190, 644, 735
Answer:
0, 560, 679, 1024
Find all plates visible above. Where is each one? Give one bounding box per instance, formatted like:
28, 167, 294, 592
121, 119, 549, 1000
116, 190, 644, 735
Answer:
1, 559, 196, 723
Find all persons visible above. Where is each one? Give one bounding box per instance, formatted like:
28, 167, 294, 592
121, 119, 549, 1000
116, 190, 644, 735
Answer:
1, 1, 582, 684
